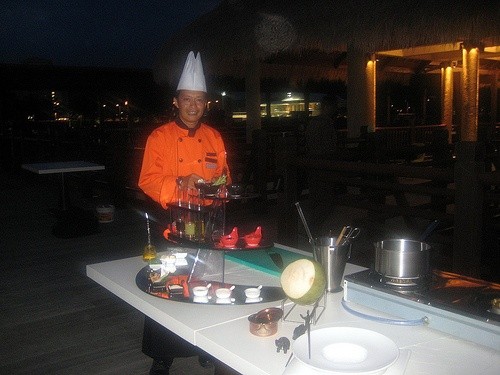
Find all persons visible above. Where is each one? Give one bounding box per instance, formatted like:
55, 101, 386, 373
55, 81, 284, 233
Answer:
137, 51, 232, 375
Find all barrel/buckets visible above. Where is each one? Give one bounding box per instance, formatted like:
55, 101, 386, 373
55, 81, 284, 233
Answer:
96, 204, 115, 224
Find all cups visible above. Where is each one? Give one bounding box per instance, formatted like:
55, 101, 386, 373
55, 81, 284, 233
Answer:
309, 236, 351, 295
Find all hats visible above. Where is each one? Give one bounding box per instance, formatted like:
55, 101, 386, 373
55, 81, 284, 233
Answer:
177, 50, 208, 94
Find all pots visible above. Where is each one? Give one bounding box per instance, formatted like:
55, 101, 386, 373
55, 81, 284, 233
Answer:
370, 239, 432, 280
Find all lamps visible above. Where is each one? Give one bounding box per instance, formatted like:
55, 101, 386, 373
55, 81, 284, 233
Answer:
441, 60, 457, 71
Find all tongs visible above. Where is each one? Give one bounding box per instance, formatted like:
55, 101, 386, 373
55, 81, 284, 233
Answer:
340, 226, 359, 245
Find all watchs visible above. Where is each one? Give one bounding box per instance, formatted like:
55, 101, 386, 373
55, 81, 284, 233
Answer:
176, 176, 183, 188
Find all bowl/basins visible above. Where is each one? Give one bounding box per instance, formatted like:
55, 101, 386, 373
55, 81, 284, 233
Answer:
193, 286, 261, 299
193, 296, 263, 304
193, 182, 221, 196
226, 183, 246, 197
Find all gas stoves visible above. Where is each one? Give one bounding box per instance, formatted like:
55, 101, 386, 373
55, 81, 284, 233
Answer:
343, 267, 500, 351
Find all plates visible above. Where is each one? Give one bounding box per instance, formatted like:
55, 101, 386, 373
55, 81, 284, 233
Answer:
292, 326, 399, 372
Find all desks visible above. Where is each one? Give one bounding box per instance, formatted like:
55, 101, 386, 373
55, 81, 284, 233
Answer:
86, 242, 500, 375
21, 160, 105, 232
